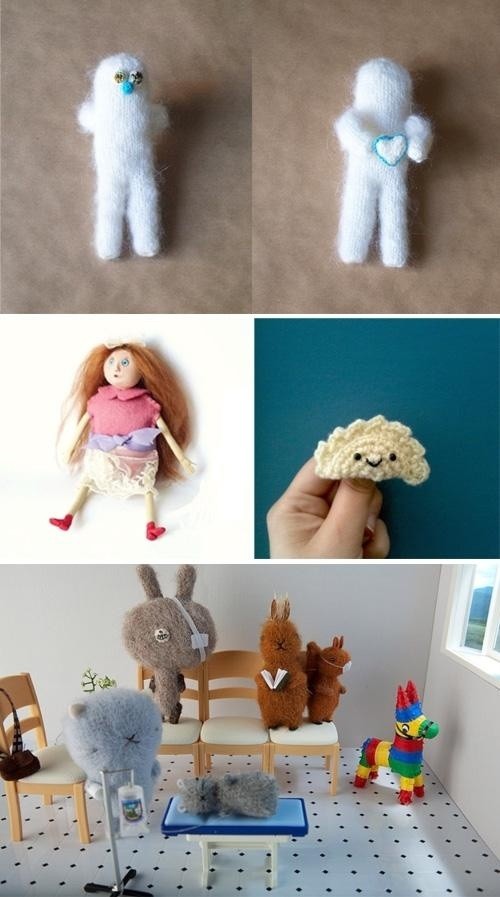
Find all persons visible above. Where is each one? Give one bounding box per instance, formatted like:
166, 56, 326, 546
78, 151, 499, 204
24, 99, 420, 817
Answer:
266, 454, 391, 558
50, 341, 198, 541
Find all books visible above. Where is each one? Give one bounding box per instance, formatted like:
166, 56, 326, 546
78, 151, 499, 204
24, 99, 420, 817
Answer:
260, 668, 290, 690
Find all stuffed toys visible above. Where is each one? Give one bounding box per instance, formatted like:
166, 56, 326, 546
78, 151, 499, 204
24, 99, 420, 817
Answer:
63, 687, 162, 839
313, 414, 430, 487
306, 636, 352, 724
353, 680, 439, 804
254, 598, 307, 730
332, 58, 434, 267
177, 770, 278, 816
120, 563, 216, 723
77, 52, 172, 259
